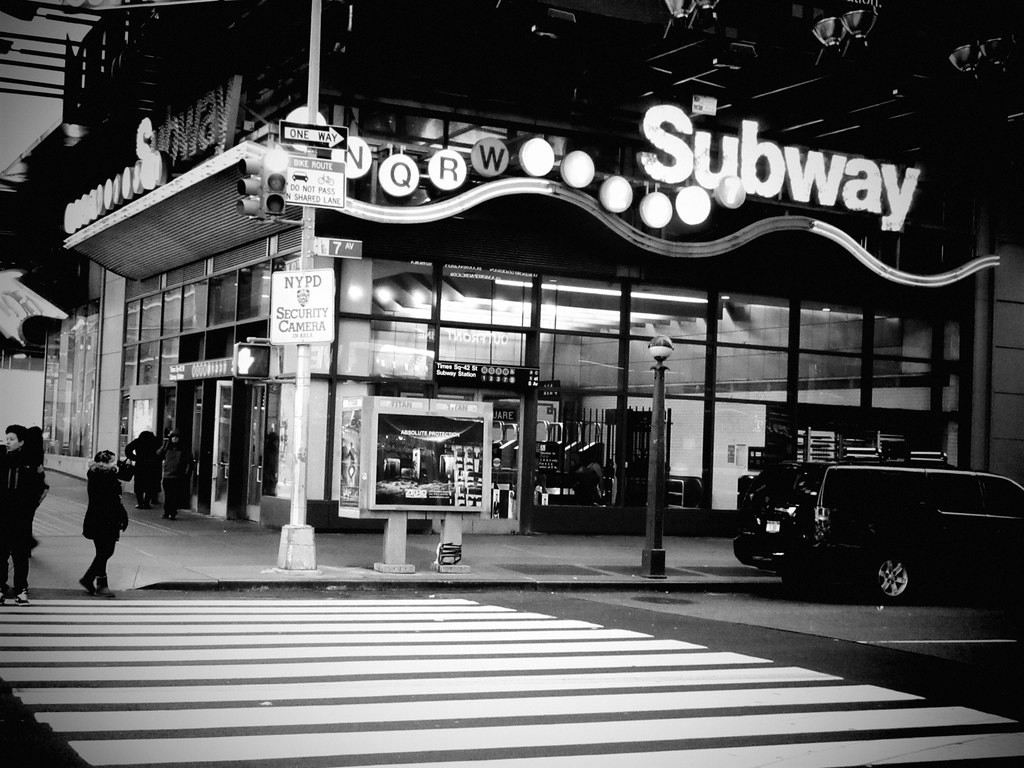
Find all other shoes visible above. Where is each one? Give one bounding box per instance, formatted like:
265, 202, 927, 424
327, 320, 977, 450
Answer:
143, 503, 153, 509
134, 505, 143, 508
151, 499, 161, 505
169, 514, 177, 520
161, 513, 168, 519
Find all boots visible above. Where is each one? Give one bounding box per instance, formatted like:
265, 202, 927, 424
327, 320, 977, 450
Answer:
79, 569, 96, 597
95, 576, 115, 598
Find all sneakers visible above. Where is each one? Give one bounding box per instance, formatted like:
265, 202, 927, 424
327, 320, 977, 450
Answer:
0, 590, 5, 605
15, 589, 30, 606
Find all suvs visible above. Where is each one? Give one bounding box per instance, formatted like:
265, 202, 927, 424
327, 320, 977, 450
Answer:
732, 451, 1024, 603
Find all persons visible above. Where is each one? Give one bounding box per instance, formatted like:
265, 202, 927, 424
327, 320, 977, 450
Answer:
78, 450, 129, 598
125, 428, 190, 521
0, 424, 49, 603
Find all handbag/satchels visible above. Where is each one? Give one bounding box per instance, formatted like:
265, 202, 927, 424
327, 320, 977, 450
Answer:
115, 457, 136, 482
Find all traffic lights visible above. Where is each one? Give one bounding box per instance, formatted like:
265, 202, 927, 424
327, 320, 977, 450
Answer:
237, 149, 263, 220
232, 342, 272, 380
263, 168, 287, 215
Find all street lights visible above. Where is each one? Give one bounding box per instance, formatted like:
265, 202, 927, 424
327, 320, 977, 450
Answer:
641, 334, 676, 578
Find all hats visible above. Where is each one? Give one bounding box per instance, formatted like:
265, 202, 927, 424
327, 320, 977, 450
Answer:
138, 431, 154, 443
167, 429, 182, 436
